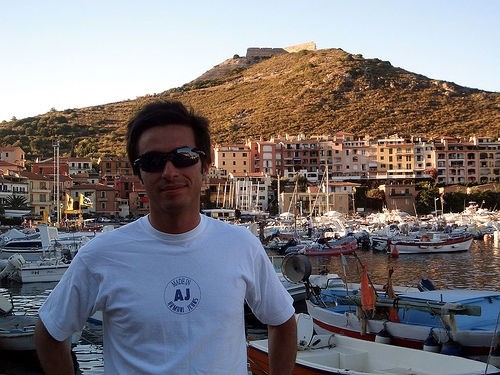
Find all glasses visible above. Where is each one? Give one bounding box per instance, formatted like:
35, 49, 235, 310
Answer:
133, 145, 205, 174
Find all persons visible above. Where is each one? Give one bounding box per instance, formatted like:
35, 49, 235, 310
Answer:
70, 222, 75, 233
34, 99, 297, 375
224, 214, 458, 249
304, 217, 312, 240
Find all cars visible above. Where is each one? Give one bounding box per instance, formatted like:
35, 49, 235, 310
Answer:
97, 216, 140, 222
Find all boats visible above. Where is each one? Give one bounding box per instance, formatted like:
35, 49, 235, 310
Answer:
0, 163, 482, 375
